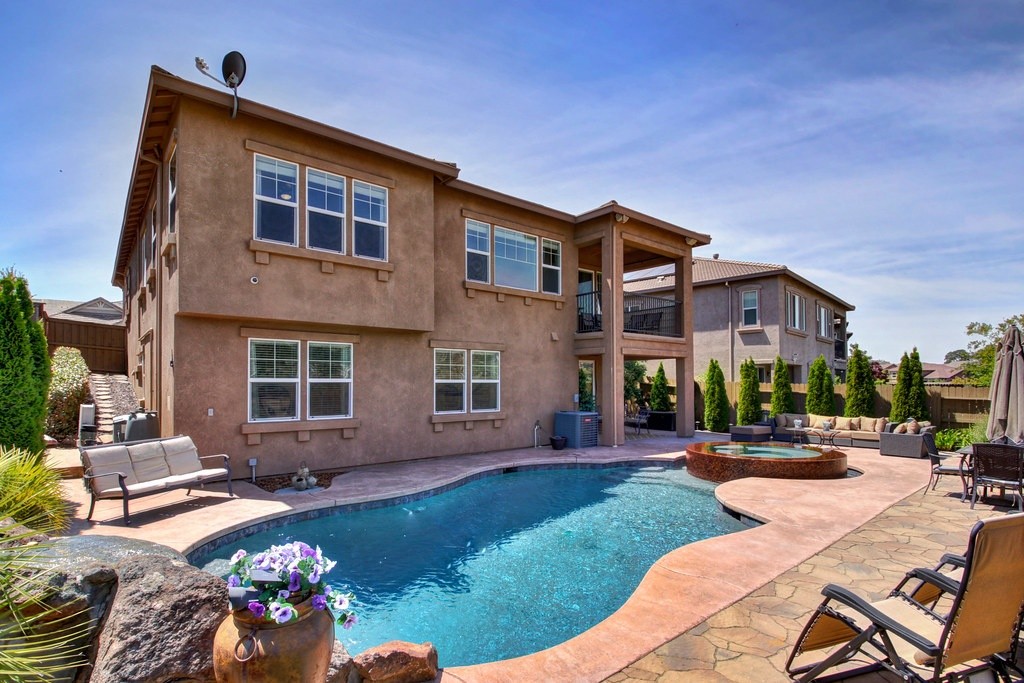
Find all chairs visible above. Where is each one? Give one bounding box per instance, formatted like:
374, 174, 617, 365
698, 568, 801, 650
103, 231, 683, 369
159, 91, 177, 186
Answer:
897, 553, 1023, 650
639, 312, 662, 335
594, 313, 600, 330
955, 445, 1024, 506
581, 312, 594, 331
921, 431, 973, 496
626, 314, 646, 333
785, 512, 1023, 683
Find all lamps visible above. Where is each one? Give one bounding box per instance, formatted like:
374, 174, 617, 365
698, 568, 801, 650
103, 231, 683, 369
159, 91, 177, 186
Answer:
280, 185, 291, 199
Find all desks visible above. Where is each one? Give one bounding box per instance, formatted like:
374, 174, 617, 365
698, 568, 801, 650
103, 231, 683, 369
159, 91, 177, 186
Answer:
953, 441, 974, 502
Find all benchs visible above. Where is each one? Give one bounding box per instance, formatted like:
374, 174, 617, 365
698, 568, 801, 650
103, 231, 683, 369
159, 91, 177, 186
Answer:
78, 434, 235, 518
624, 407, 650, 435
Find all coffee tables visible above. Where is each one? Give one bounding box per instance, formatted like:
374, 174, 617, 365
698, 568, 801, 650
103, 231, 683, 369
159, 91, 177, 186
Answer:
814, 429, 842, 450
783, 426, 812, 445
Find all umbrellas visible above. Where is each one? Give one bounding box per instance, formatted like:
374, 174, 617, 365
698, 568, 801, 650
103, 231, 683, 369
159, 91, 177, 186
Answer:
985, 324, 1024, 501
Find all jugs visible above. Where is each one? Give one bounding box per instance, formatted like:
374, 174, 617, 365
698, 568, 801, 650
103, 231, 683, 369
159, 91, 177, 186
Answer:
289, 460, 317, 489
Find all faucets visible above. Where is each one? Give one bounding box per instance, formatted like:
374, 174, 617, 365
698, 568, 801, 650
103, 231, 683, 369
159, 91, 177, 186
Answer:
534, 420, 542, 429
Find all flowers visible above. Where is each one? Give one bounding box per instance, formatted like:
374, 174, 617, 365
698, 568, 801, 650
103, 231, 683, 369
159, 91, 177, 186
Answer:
225, 540, 358, 629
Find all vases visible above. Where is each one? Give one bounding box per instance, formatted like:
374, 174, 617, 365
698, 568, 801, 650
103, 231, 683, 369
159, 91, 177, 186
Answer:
212, 591, 334, 682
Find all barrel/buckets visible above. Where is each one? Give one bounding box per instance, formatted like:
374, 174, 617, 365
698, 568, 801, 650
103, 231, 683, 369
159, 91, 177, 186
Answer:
550, 436, 567, 450
822, 422, 832, 431
794, 419, 802, 429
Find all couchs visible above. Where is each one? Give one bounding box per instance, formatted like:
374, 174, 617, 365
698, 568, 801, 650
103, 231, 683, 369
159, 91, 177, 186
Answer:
770, 413, 851, 446
878, 423, 936, 459
727, 421, 773, 443
851, 420, 890, 448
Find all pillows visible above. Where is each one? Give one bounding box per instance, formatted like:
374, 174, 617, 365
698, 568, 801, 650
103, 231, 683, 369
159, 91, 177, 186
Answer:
834, 417, 851, 429
813, 415, 831, 428
892, 422, 907, 433
843, 416, 860, 431
775, 413, 787, 427
785, 415, 800, 427
874, 416, 887, 432
860, 416, 878, 433
906, 420, 920, 434
